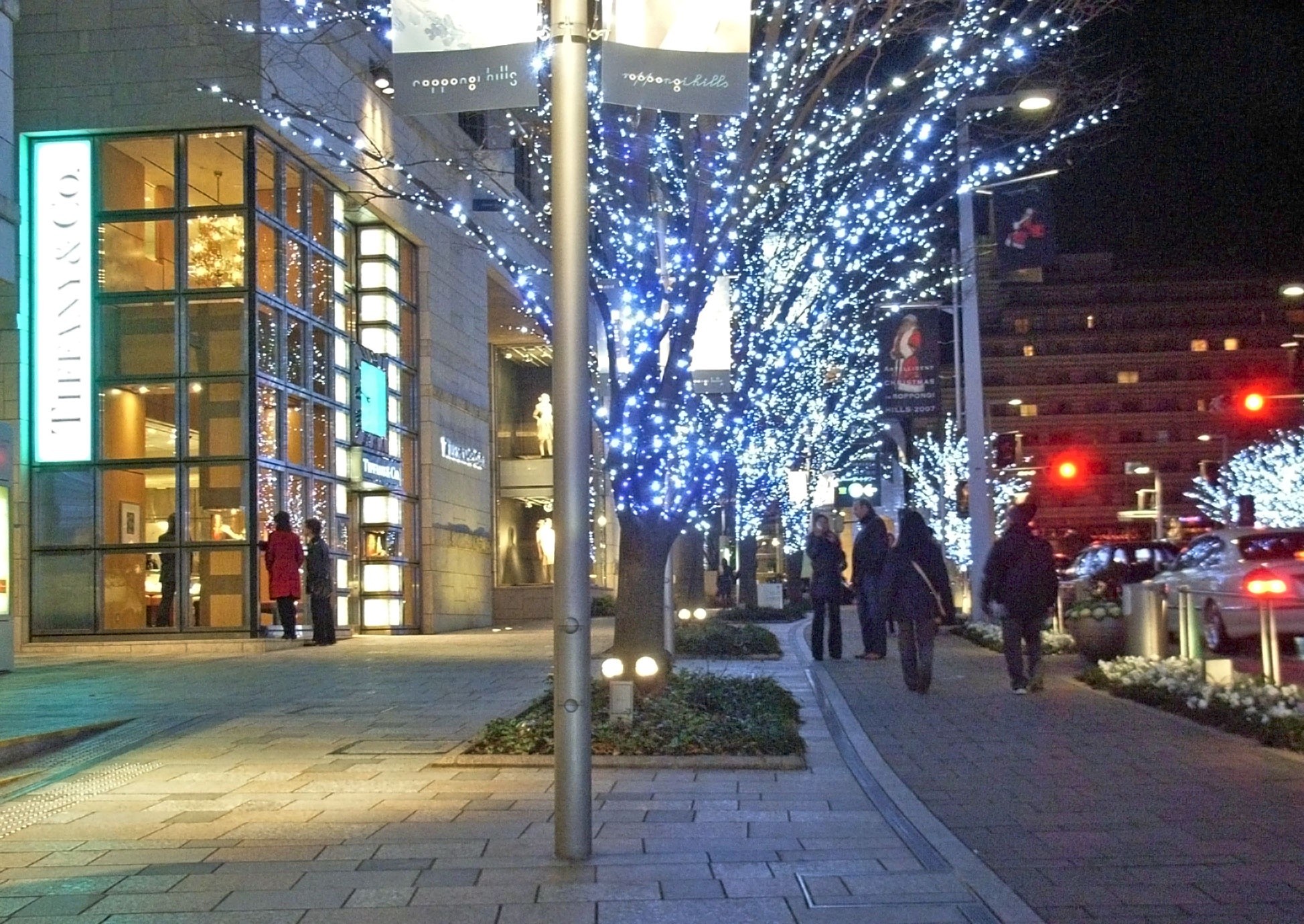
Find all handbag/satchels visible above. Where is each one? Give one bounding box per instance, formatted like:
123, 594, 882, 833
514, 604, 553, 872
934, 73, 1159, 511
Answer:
931, 591, 948, 625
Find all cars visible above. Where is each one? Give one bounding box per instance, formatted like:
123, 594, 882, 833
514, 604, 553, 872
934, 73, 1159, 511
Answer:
1141, 527, 1304, 655
1061, 540, 1185, 602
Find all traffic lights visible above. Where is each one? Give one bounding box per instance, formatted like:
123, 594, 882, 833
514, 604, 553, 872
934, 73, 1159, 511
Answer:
1210, 392, 1266, 417
996, 430, 1022, 467
1056, 462, 1111, 478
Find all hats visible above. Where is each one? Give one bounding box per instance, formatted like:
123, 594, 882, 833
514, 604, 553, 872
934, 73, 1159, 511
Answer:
1009, 503, 1036, 525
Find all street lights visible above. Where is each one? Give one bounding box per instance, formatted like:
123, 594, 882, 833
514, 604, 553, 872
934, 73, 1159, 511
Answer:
986, 398, 1021, 539
1132, 466, 1163, 542
1197, 434, 1231, 529
955, 85, 1061, 626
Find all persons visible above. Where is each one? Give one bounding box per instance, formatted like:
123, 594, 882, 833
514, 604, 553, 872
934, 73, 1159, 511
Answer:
154, 514, 194, 628
127, 514, 136, 535
533, 393, 554, 457
851, 498, 889, 660
212, 514, 243, 540
265, 510, 304, 640
536, 518, 555, 584
304, 517, 337, 646
840, 577, 856, 604
890, 314, 927, 394
876, 511, 956, 695
1004, 206, 1048, 273
979, 503, 1059, 695
147, 554, 162, 571
713, 558, 741, 610
805, 514, 849, 660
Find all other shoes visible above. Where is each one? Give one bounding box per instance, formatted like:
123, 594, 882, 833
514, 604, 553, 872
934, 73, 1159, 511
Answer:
855, 651, 885, 659
1031, 678, 1044, 691
303, 639, 325, 646
812, 650, 823, 660
1011, 682, 1027, 694
282, 632, 297, 640
326, 636, 336, 643
830, 649, 842, 658
918, 679, 931, 692
904, 676, 919, 691
